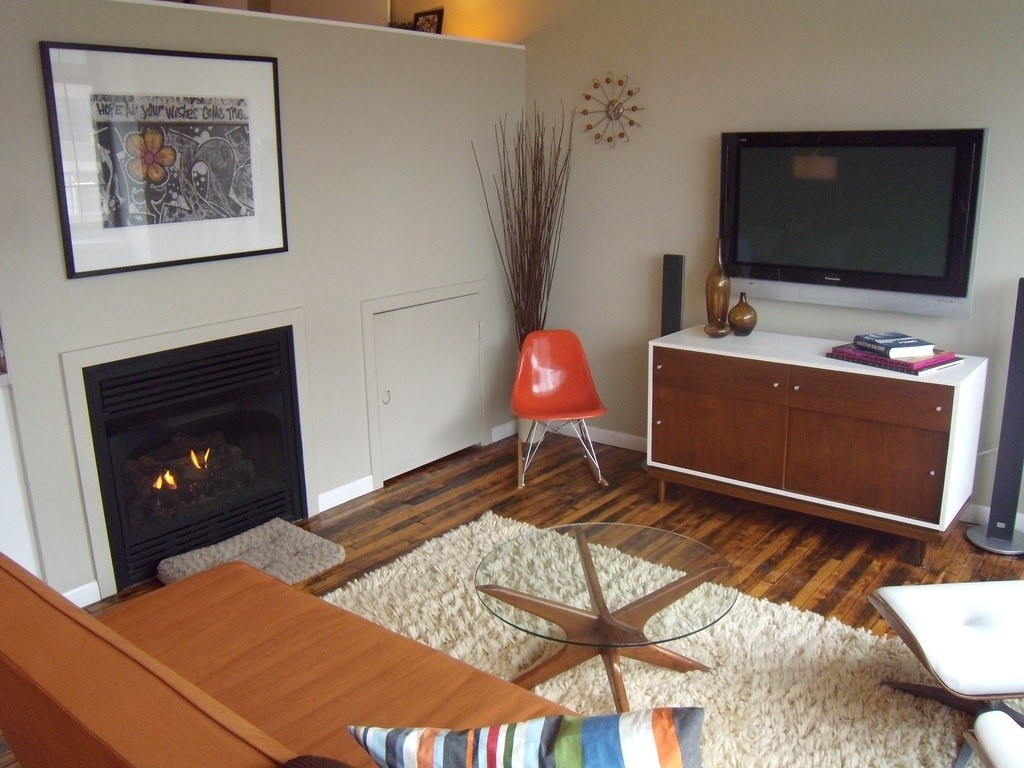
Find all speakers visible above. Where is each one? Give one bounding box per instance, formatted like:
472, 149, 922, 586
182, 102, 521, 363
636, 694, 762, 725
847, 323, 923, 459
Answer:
967, 278, 1024, 554
661, 254, 685, 337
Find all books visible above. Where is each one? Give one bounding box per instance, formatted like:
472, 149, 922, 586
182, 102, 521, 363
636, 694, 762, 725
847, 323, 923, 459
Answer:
825, 331, 966, 376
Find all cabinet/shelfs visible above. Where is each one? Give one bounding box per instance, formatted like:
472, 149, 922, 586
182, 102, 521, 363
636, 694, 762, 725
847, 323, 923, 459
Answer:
641, 321, 990, 568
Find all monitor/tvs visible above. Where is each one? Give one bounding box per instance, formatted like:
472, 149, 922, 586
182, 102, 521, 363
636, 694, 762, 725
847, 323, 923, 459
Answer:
723, 127, 990, 320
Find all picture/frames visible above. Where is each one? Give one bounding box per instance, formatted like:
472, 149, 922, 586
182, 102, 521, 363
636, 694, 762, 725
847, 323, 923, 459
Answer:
38, 41, 288, 280
413, 7, 444, 35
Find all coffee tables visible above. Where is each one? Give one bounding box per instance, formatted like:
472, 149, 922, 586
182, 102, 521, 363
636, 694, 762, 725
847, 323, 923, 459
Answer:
473, 523, 740, 712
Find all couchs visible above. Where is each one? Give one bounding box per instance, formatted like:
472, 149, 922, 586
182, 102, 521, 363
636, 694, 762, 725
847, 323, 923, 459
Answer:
1, 550, 580, 766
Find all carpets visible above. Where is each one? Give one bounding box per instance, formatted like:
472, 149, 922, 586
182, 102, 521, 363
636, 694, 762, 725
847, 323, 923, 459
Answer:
315, 510, 1024, 767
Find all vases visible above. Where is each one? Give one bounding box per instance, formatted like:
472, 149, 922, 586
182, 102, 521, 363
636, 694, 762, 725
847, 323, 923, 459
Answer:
703, 237, 730, 339
521, 418, 548, 443
727, 291, 757, 336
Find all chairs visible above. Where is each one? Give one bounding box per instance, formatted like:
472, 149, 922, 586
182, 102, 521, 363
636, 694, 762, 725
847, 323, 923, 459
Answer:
511, 329, 609, 489
868, 579, 1024, 766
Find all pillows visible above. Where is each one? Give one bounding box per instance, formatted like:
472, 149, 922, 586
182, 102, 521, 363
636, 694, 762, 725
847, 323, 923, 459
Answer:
346, 707, 705, 766
